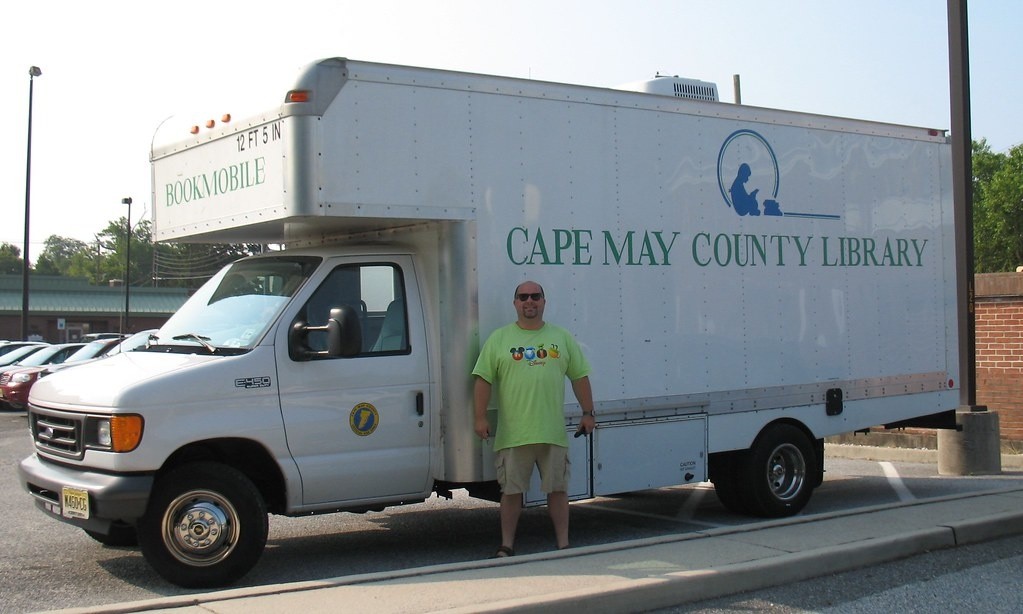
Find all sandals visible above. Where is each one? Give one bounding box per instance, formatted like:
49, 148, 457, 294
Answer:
555, 544, 576, 550
489, 546, 514, 559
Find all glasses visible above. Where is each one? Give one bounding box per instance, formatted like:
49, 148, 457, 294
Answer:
516, 293, 541, 301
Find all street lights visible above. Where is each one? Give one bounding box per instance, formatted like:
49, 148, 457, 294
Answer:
19, 65, 43, 342
120, 195, 132, 331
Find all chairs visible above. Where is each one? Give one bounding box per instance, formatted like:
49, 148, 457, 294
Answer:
309, 298, 409, 352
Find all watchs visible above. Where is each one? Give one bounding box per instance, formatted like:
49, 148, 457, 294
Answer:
583, 410, 595, 416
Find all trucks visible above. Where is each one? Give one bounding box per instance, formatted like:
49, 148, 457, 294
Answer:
17, 56, 964, 590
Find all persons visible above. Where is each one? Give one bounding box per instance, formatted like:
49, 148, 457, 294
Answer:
472, 282, 596, 558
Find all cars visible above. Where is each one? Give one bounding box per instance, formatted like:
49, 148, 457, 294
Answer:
0, 331, 134, 412
